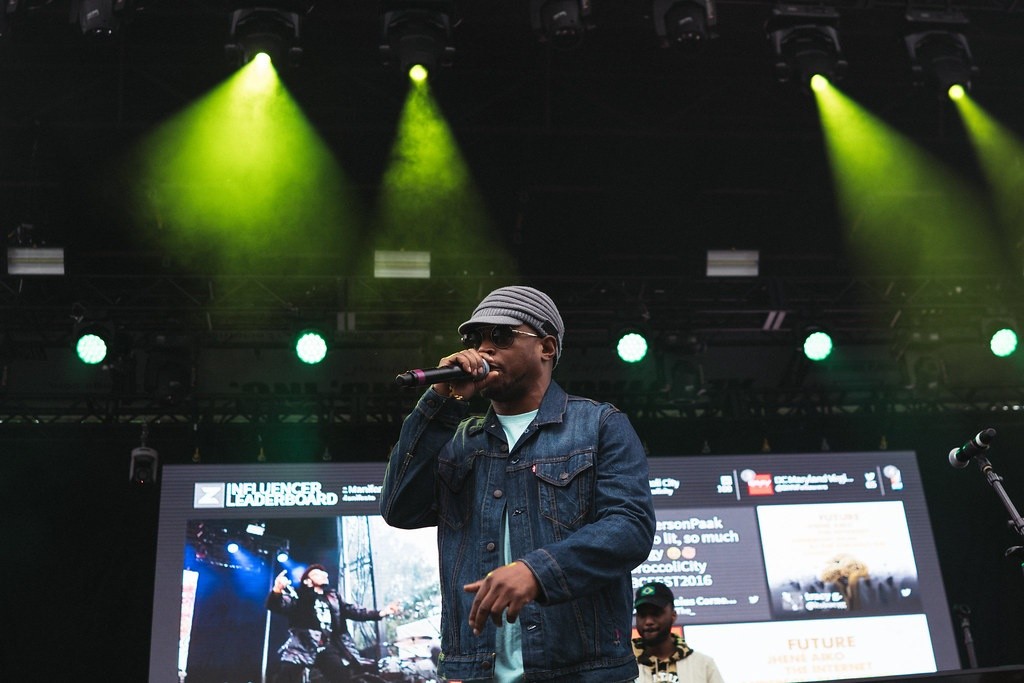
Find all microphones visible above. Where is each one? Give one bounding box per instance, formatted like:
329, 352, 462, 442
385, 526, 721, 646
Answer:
396, 358, 490, 389
948, 427, 997, 469
284, 585, 300, 601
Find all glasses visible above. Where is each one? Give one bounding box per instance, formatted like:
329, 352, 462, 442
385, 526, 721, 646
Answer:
460, 324, 540, 351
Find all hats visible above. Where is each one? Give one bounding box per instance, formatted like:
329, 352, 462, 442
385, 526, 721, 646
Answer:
634, 582, 675, 610
458, 285, 565, 371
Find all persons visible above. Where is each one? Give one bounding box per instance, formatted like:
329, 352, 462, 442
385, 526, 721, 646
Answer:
631, 582, 726, 683
380, 286, 656, 683
267, 564, 390, 683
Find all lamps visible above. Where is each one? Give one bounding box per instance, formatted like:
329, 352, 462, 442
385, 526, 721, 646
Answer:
530, 0, 598, 53
894, 0, 986, 122
227, 0, 301, 75
652, 0, 716, 54
764, 0, 849, 101
67, 306, 1021, 367
68, 0, 124, 43
382, 0, 458, 90
